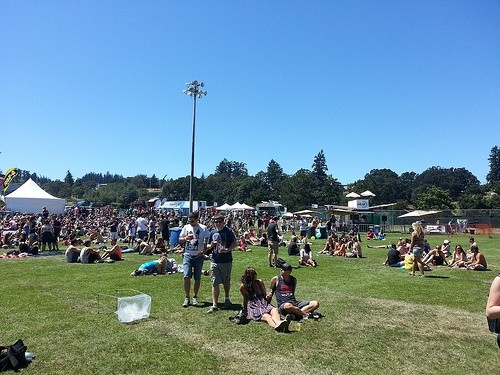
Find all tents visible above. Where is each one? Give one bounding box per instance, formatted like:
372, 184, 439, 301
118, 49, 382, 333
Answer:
3, 178, 66, 216
217, 202, 255, 215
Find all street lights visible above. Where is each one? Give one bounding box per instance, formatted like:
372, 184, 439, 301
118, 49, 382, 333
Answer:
182, 79, 207, 217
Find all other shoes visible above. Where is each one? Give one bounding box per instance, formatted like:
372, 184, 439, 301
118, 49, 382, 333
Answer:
274, 314, 291, 333
408, 271, 415, 276
207, 305, 221, 313
225, 300, 234, 311
308, 311, 322, 319
292, 314, 302, 320
182, 297, 190, 306
192, 298, 198, 306
420, 275, 425, 278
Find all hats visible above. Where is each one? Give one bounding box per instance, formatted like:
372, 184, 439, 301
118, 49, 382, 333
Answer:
279, 263, 292, 274
392, 244, 396, 248
443, 240, 451, 245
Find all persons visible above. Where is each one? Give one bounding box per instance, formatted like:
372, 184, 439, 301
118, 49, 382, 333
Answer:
206, 214, 237, 313
318, 215, 362, 258
300, 216, 319, 239
436, 219, 440, 228
0, 206, 225, 276
423, 238, 487, 270
269, 264, 319, 320
384, 237, 430, 270
288, 232, 313, 266
239, 268, 291, 331
367, 245, 392, 248
225, 212, 273, 251
449, 219, 453, 225
486, 273, 500, 348
266, 217, 286, 267
409, 223, 425, 277
178, 212, 210, 307
367, 229, 386, 240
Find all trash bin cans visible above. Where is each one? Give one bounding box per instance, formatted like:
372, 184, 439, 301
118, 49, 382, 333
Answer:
306, 226, 316, 239
169, 227, 183, 248
316, 227, 326, 239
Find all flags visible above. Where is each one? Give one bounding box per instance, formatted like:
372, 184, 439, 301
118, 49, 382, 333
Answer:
3, 168, 18, 193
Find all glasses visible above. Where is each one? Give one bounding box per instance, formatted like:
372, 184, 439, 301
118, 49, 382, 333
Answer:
187, 220, 195, 223
457, 248, 461, 249
214, 221, 223, 223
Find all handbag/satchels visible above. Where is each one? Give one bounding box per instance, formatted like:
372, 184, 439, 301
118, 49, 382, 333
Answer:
0, 338, 27, 371
